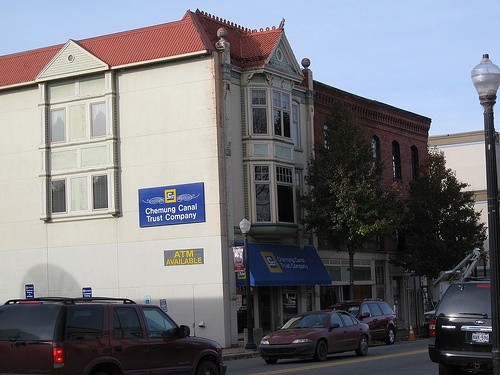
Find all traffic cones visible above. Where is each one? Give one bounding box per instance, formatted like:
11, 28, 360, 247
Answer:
408, 325, 416, 341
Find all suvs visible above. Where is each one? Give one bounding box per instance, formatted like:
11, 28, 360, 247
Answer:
0, 298, 226, 375
328, 298, 398, 345
427, 280, 500, 375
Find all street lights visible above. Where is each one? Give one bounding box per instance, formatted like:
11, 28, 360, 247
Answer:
469, 54, 500, 347
238, 217, 257, 350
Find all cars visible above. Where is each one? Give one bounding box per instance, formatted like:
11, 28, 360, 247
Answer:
259, 310, 371, 364
